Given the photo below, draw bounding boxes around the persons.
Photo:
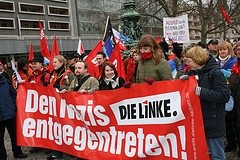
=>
[180,46,230,160]
[123,35,173,88]
[0,35,240,160]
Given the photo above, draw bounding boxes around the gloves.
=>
[123,81,132,88]
[142,78,153,85]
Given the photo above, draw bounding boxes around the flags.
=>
[27,43,35,61]
[49,34,61,74]
[112,28,127,46]
[77,38,85,56]
[221,7,232,25]
[39,20,51,60]
[85,42,103,78]
[102,15,114,42]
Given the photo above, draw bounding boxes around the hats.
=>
[29,57,43,63]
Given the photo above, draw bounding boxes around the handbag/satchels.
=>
[225,95,234,111]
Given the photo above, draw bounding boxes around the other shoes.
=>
[47,154,62,160]
[15,153,27,158]
